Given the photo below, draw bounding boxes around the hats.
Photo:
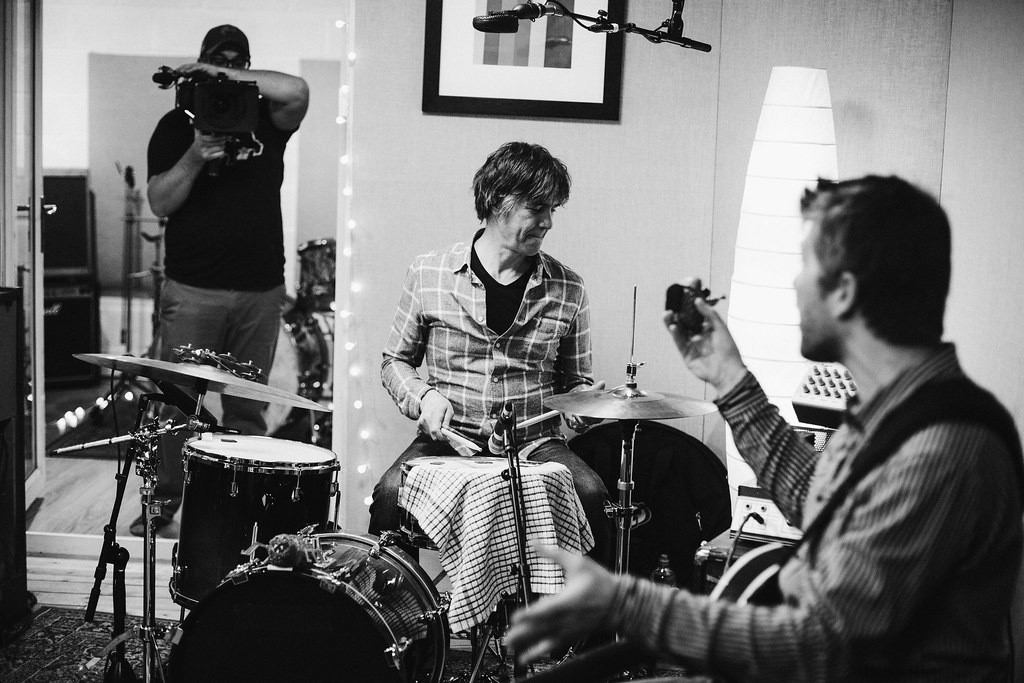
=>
[201,24,249,53]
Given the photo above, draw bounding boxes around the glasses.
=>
[208,56,251,70]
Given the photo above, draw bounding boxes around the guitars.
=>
[505,541,799,683]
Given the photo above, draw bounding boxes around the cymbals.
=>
[72,351,335,414]
[540,384,718,421]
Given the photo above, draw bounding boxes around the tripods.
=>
[66,379,211,683]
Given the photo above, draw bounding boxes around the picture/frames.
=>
[421,0,625,121]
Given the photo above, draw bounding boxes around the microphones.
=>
[473,4,556,33]
[489,400,514,454]
[151,72,173,84]
[152,378,218,425]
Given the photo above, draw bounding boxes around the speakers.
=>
[40,167,102,385]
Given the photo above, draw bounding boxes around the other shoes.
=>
[129,507,174,537]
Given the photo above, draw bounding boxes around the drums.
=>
[295,238,340,308]
[199,290,336,436]
[400,450,567,551]
[163,530,450,683]
[170,431,341,613]
[563,417,731,589]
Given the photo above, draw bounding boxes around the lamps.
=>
[726,66,838,518]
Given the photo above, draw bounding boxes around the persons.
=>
[369,142,618,573]
[500,173,1024,683]
[125,27,312,533]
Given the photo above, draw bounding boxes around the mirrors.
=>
[6,0,356,559]
